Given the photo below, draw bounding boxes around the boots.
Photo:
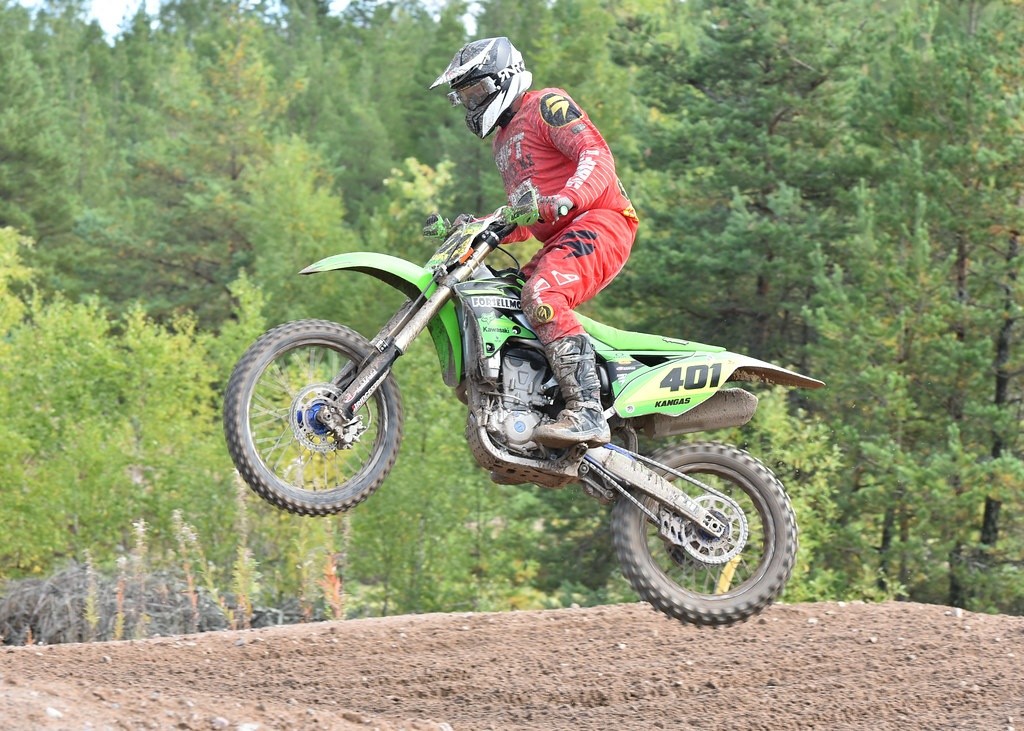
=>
[489,470,529,485]
[534,333,611,450]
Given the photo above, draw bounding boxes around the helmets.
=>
[429,36,532,138]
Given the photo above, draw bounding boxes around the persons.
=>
[430,37,640,449]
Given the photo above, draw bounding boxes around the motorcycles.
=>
[223,204,826,630]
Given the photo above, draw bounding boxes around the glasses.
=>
[447,76,499,108]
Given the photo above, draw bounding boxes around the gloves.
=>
[536,195,574,224]
[452,213,475,226]
